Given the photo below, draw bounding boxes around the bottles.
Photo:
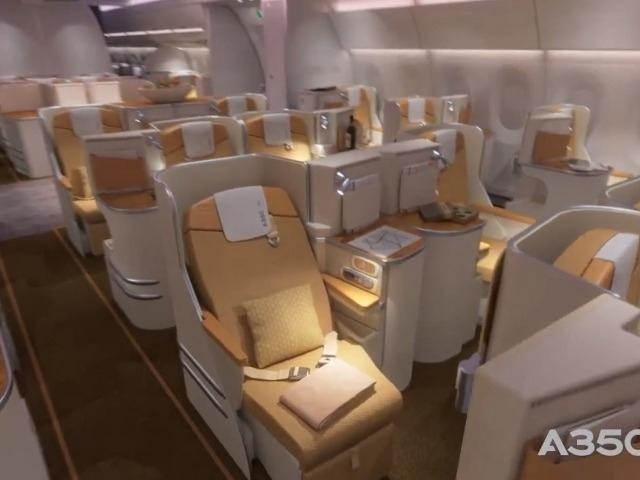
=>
[344,115,356,150]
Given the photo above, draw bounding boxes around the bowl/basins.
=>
[138,85,193,104]
